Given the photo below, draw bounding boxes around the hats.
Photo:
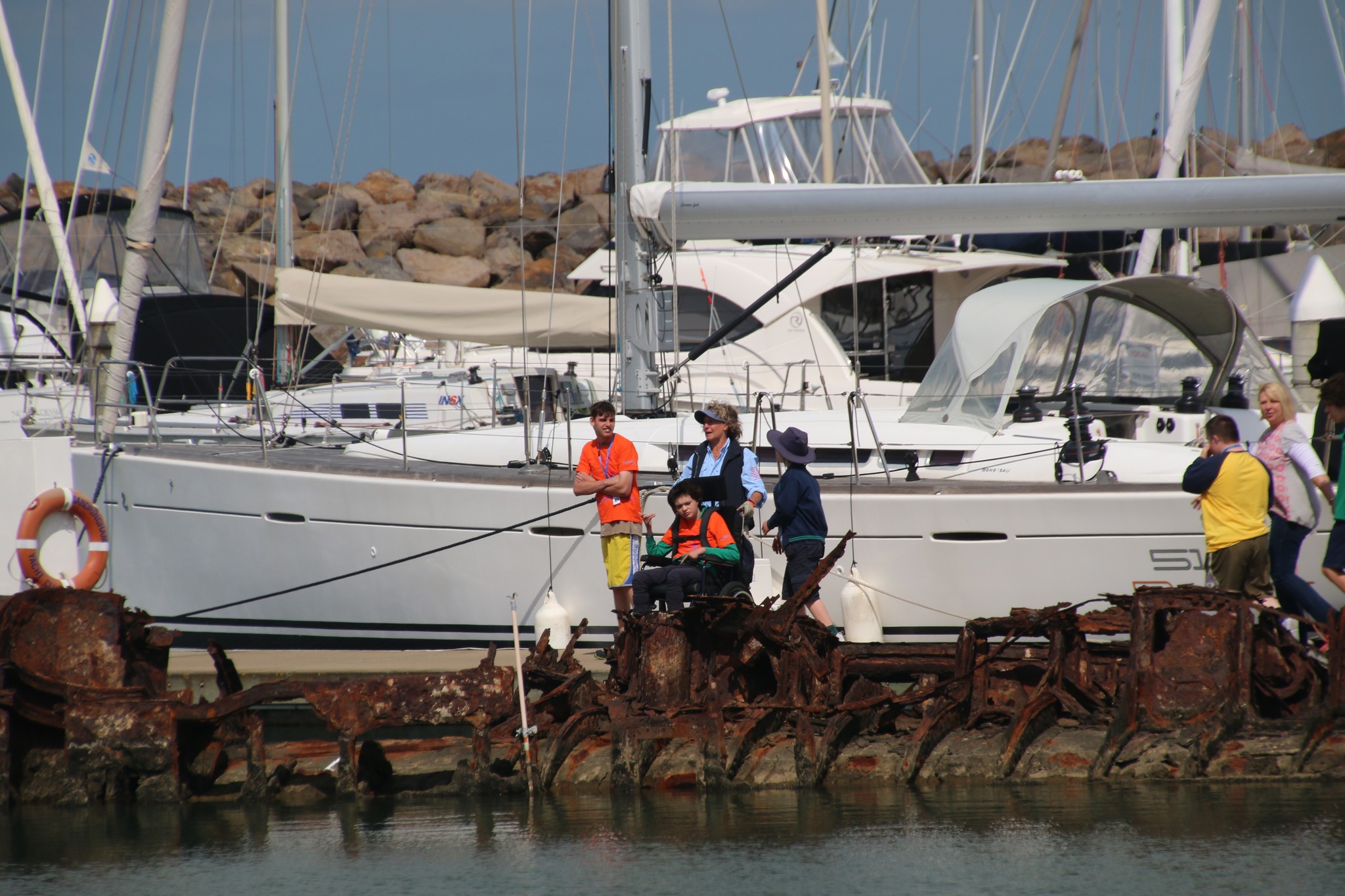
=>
[694,409,726,425]
[766,427,816,464]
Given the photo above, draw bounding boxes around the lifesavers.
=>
[15,487,109,591]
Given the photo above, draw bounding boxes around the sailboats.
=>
[0,1,1345,679]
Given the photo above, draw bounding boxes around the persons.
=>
[1183,415,1289,630]
[1317,372,1345,592]
[573,400,844,639]
[1253,381,1342,653]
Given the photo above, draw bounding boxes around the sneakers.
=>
[594,645,615,659]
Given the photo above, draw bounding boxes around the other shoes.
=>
[1309,626,1330,652]
[836,630,846,642]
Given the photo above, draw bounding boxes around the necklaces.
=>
[711,451,721,457]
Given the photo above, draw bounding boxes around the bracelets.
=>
[1329,495,1336,504]
[747,498,757,507]
